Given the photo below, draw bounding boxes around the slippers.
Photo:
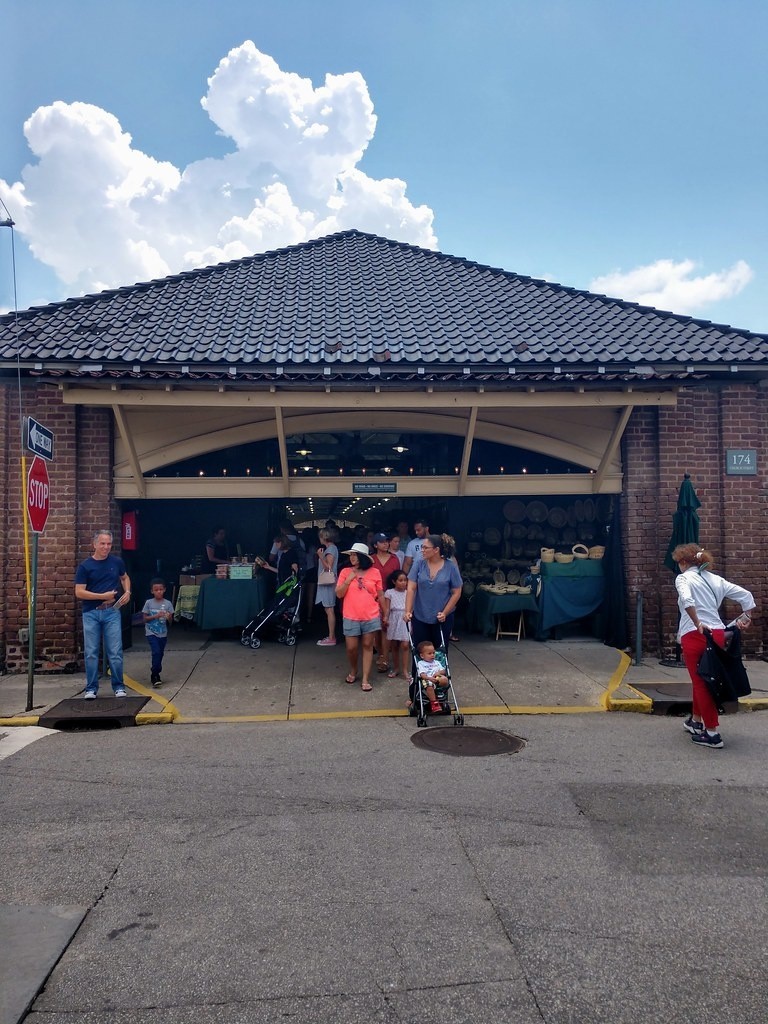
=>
[361,683,372,691]
[346,672,356,683]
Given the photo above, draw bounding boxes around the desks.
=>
[538,560,609,640]
[194,578,272,631]
[476,586,538,642]
[174,586,201,622]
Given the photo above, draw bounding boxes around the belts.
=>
[95,603,113,610]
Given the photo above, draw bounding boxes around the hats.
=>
[341,543,375,564]
[371,533,388,546]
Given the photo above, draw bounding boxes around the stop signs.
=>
[27,455,52,533]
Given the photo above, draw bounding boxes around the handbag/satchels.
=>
[318,566,336,586]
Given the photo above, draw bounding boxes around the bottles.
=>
[230,557,238,565]
[238,553,249,564]
[159,604,166,623]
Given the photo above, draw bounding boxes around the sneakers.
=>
[684,716,703,735]
[115,689,126,697]
[691,728,724,748]
[84,691,97,699]
[316,637,336,646]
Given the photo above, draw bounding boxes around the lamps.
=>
[392,434,410,452]
[295,433,312,455]
[299,454,313,471]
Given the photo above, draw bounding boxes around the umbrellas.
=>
[663,479,701,572]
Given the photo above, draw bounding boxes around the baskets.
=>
[541,547,555,562]
[555,552,574,564]
[572,543,589,558]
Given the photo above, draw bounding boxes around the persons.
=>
[201,525,229,574]
[334,543,387,691]
[141,578,174,686]
[338,520,459,672]
[262,518,338,646]
[417,641,448,712]
[672,543,756,749]
[75,530,130,698]
[385,569,411,682]
[403,533,463,707]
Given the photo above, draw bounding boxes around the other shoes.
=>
[404,675,411,681]
[152,675,162,686]
[449,636,460,642]
[377,656,391,672]
[431,700,442,712]
[388,671,400,677]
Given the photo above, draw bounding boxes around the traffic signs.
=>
[28,416,54,461]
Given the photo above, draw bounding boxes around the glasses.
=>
[421,546,437,550]
[358,578,362,588]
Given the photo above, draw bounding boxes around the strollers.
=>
[408,620,465,728]
[240,568,307,649]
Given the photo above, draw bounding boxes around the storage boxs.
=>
[179,574,211,585]
[216,562,259,579]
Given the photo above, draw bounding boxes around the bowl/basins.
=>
[482,584,519,595]
[255,555,265,566]
[517,587,531,595]
[215,564,228,579]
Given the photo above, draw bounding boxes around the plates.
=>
[520,573,530,587]
[493,571,505,583]
[507,569,521,584]
[484,499,596,545]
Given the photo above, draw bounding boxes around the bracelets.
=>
[698,623,701,628]
[126,591,131,594]
